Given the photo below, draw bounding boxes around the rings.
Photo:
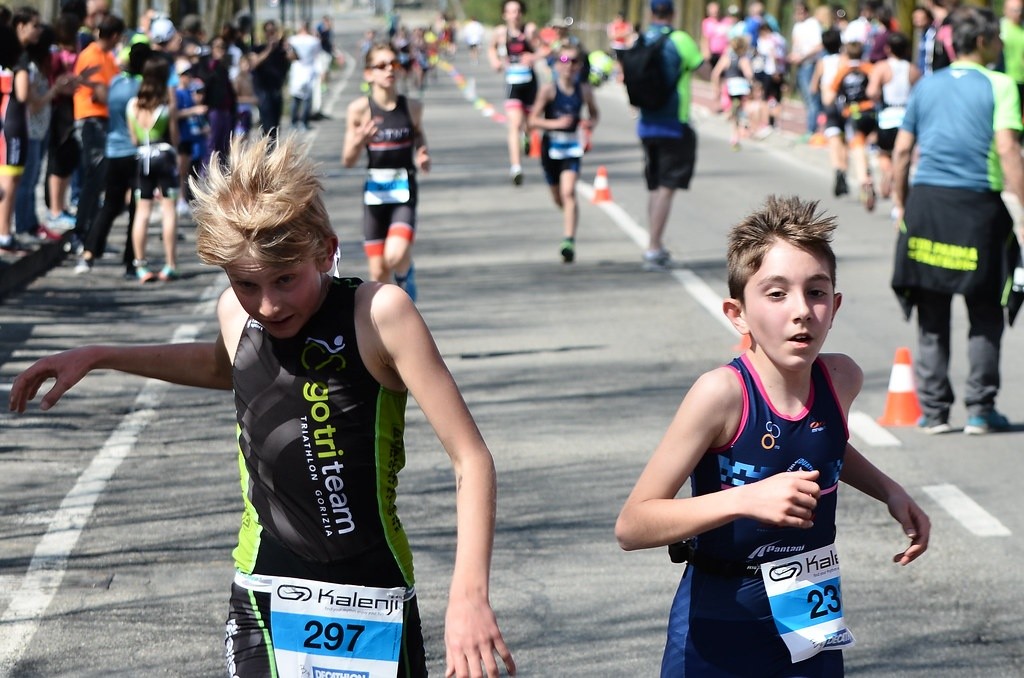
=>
[61,79,68,84]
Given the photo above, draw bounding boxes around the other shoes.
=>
[0,204,78,257]
[917,414,951,435]
[862,180,874,211]
[394,259,417,302]
[835,169,848,197]
[964,409,1011,435]
[559,240,576,262]
[640,249,678,272]
[74,260,95,276]
[135,266,178,283]
[511,166,523,185]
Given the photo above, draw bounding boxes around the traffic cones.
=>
[527,131,542,159]
[875,347,924,424]
[589,165,615,204]
[729,332,752,353]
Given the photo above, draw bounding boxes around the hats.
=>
[652,0,674,13]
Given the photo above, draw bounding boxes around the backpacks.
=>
[616,29,683,107]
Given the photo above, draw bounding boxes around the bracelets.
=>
[0,0,337,284]
[49,86,59,99]
[284,47,294,54]
[76,75,84,82]
[133,258,148,267]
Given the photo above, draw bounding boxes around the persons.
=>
[700,0,1024,211]
[340,41,431,302]
[7,127,517,678]
[637,0,711,271]
[357,0,484,94]
[614,195,931,678]
[889,7,1024,435]
[488,0,600,263]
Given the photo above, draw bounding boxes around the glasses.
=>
[370,58,401,70]
[559,55,579,64]
